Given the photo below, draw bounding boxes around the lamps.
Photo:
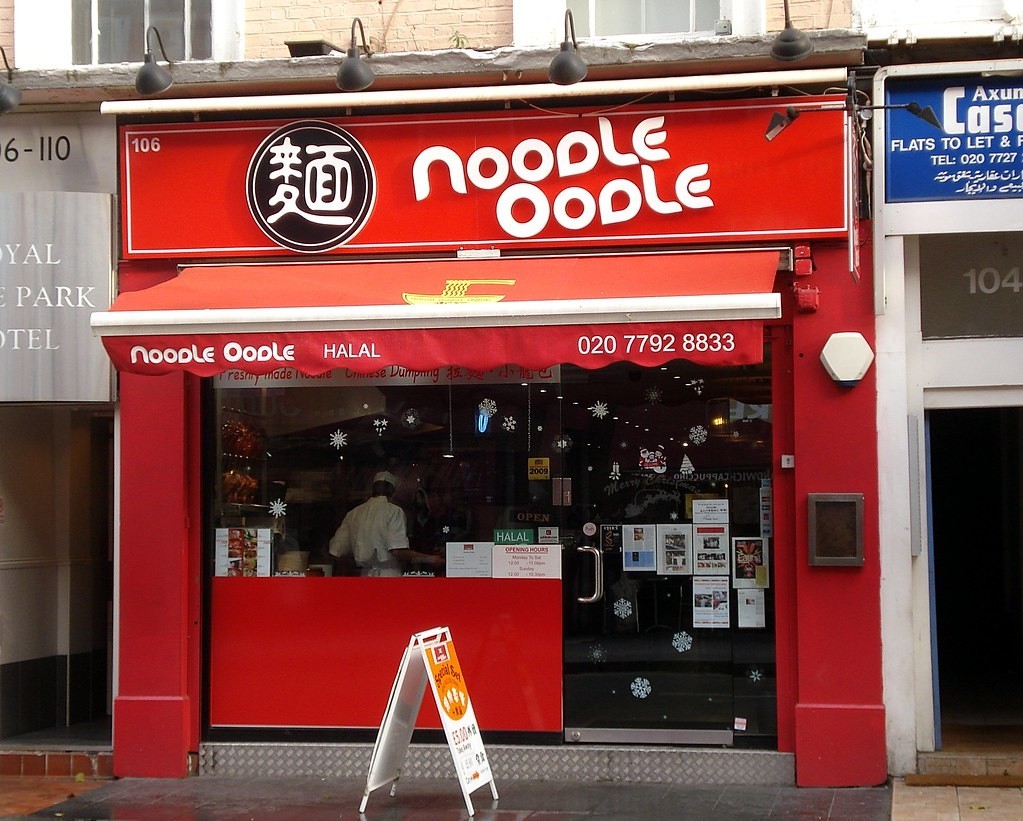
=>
[134,24,175,96]
[335,17,376,91]
[770,0,814,61]
[547,7,588,85]
[0,45,23,116]
[786,102,950,134]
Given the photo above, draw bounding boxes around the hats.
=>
[374,471,397,488]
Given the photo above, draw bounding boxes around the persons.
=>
[328,471,445,576]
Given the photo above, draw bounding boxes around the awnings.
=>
[96,253,787,375]
